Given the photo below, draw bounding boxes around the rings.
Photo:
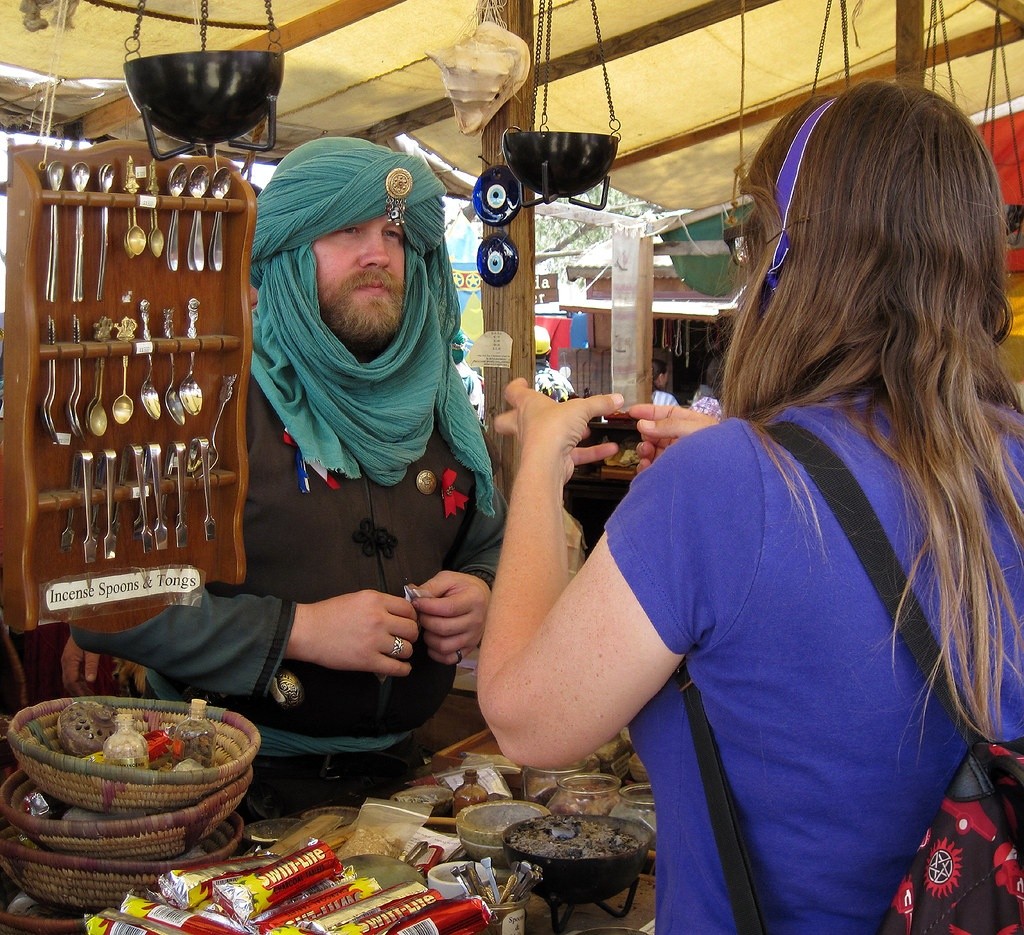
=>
[456,650,463,663]
[387,636,404,658]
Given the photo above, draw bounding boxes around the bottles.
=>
[545,773,622,815]
[452,769,487,819]
[607,782,657,851]
[103,712,149,770]
[585,724,650,782]
[522,760,590,807]
[172,698,218,769]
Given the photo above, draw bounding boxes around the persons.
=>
[478,77,1024,935]
[58,138,510,856]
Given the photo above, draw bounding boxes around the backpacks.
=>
[677,415,1024,935]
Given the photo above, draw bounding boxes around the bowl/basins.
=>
[391,785,452,816]
[456,801,551,867]
[243,818,299,846]
[301,807,359,824]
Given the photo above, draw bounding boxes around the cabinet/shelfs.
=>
[0,0,257,630]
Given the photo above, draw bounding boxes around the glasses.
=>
[724,224,750,268]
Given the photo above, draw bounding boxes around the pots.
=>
[501,814,654,933]
[502,130,618,210]
[124,49,284,161]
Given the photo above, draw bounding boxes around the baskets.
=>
[0,700,261,906]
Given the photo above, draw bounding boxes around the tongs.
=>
[61,435,218,564]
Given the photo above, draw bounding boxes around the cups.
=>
[426,861,496,900]
[476,890,530,935]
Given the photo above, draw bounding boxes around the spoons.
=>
[190,374,241,481]
[42,296,204,446]
[40,161,233,302]
